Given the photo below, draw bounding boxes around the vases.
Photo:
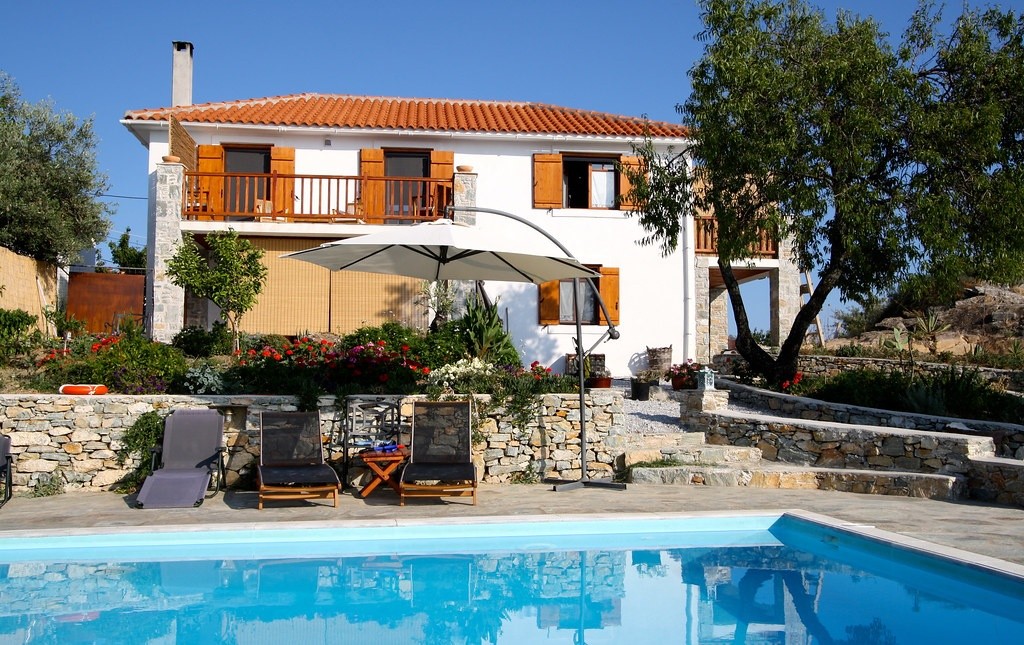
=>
[671,376,698,389]
[630,378,650,401]
[588,377,614,387]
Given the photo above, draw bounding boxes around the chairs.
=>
[255,408,342,510]
[252,198,293,221]
[334,202,364,225]
[133,408,227,510]
[399,399,478,506]
[0,433,14,509]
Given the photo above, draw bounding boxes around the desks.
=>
[187,189,223,221]
[410,195,437,225]
[356,444,412,500]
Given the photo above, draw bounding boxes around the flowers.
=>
[665,358,705,381]
